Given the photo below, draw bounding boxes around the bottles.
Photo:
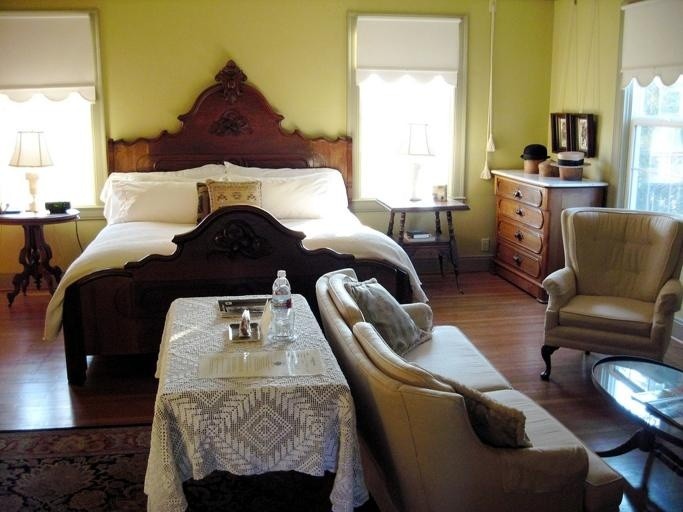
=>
[272,270,291,292]
[273,278,292,310]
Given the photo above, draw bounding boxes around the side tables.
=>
[376,198,469,293]
[1,209,81,306]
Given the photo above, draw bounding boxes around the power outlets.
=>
[481,239,490,250]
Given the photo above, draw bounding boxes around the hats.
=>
[521,144,550,160]
[549,152,591,168]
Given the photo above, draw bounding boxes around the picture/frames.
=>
[572,114,595,158]
[550,113,570,153]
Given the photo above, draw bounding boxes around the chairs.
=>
[541,207,682,380]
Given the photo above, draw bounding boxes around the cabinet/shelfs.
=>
[491,169,609,303]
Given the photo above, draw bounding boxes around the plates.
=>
[228,322,261,343]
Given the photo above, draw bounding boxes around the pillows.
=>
[354,320,454,392]
[196,183,210,223]
[228,176,321,219]
[224,161,348,210]
[437,374,531,446]
[207,180,261,216]
[111,179,206,224]
[346,276,431,355]
[100,164,223,222]
[331,273,364,327]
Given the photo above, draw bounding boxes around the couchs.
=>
[314,267,624,512]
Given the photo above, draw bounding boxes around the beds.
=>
[42,59,433,383]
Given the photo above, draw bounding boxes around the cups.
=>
[271,308,296,342]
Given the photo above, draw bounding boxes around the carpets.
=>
[0,422,391,511]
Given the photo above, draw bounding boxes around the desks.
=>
[142,294,368,502]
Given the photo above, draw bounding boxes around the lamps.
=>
[8,131,53,213]
